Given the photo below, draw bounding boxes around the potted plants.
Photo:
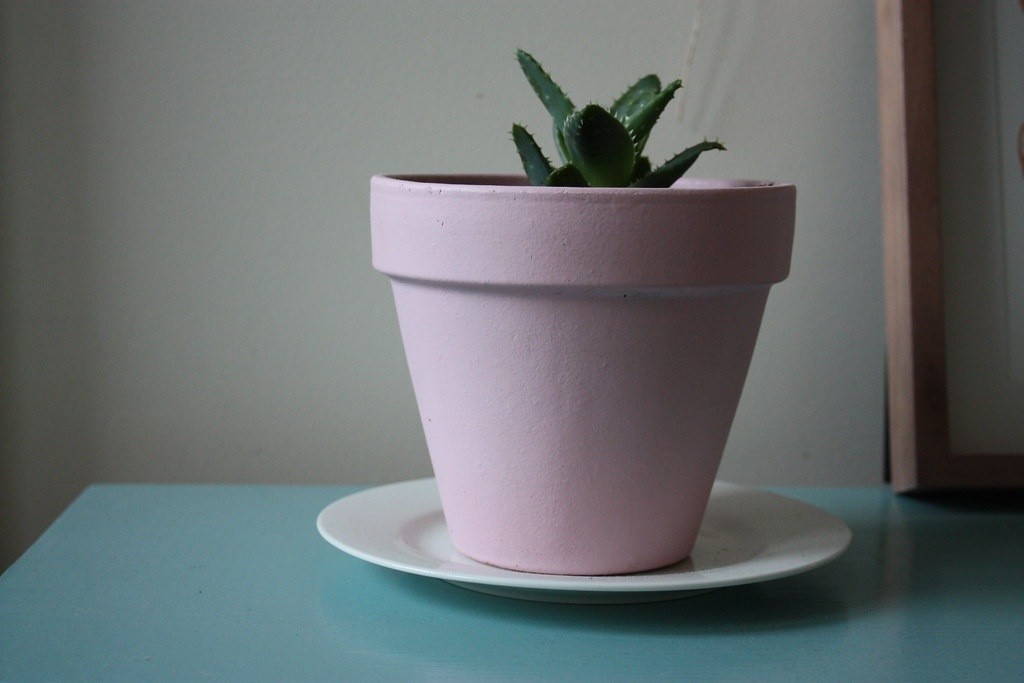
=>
[367,48,798,577]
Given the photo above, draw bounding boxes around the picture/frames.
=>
[880,0,1024,494]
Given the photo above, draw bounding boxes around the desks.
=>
[0,476,1024,683]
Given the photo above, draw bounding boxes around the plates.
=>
[317,476,855,606]
